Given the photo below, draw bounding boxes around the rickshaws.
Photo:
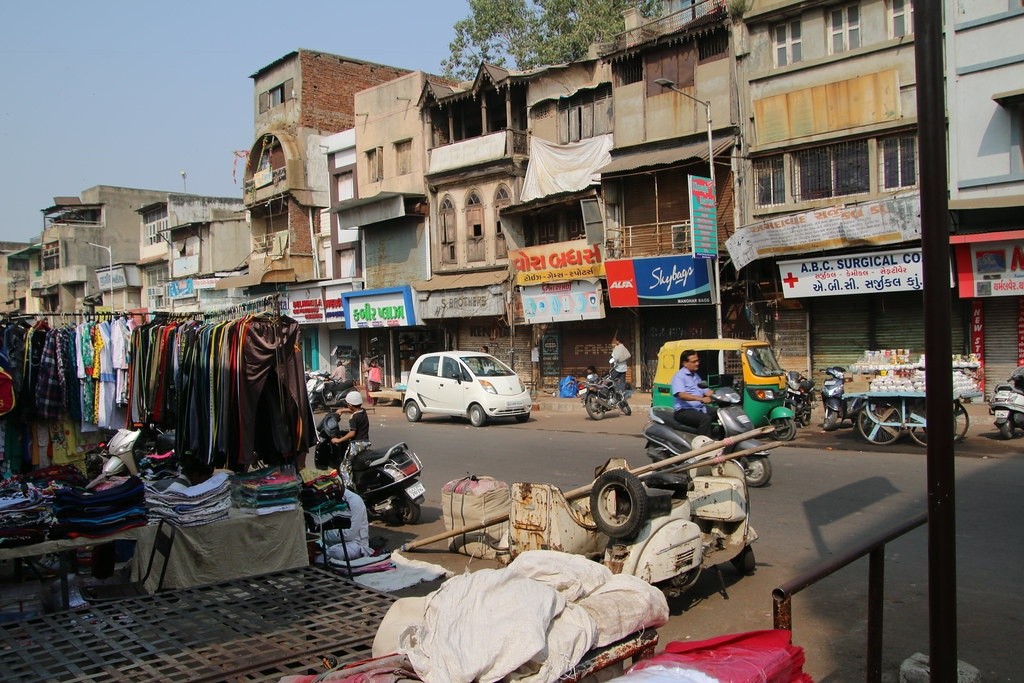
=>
[841,390,983,448]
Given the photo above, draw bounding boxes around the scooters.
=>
[603,433,761,605]
[641,383,772,488]
[987,365,1024,439]
[314,412,425,524]
[819,366,855,430]
[305,371,356,411]
[102,427,176,480]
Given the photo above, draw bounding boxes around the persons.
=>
[362,359,382,406]
[609,335,627,384]
[480,346,489,352]
[670,350,715,437]
[331,391,371,491]
[331,361,346,380]
[582,366,596,378]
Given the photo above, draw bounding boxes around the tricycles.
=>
[649,337,797,443]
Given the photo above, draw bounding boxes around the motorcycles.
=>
[779,368,815,425]
[575,357,631,420]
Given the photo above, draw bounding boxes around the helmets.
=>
[337,362,342,364]
[345,390,363,406]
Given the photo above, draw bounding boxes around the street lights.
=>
[654,79,725,378]
[80,240,114,313]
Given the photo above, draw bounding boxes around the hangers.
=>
[0,312,135,330]
[151,294,282,326]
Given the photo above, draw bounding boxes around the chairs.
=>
[79,519,176,603]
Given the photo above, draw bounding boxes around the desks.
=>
[0,504,353,610]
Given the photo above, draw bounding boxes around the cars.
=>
[402,350,533,426]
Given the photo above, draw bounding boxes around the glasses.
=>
[690,358,699,363]
[481,348,482,350]
